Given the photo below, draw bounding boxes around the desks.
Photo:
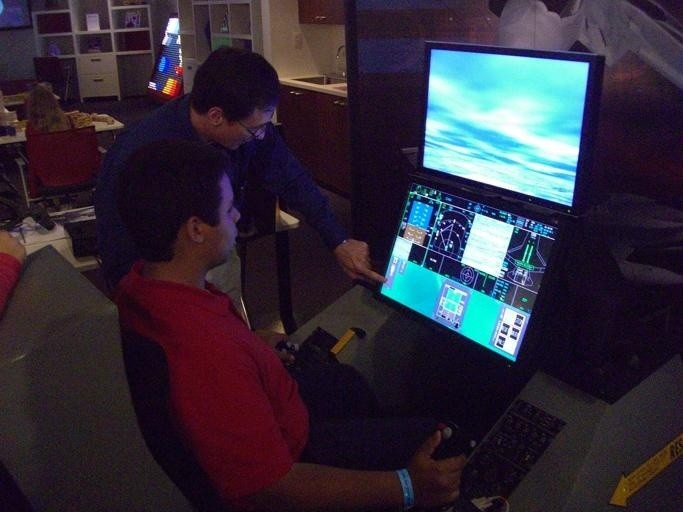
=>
[0,112,124,149]
[1,90,62,108]
[291,280,681,510]
[12,178,296,334]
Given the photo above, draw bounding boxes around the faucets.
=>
[335,45,347,78]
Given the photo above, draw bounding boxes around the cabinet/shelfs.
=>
[314,91,348,197]
[274,84,315,183]
[297,0,346,24]
[30,0,157,103]
[177,0,269,94]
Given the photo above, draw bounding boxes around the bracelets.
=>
[392,465,418,510]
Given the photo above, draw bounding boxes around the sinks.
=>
[292,76,348,85]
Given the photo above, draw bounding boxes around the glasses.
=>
[236,119,268,139]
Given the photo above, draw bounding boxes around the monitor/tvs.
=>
[373,171,564,368]
[417,40,606,214]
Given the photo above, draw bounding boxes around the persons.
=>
[0,229,25,321]
[93,44,385,330]
[103,138,467,511]
[25,82,74,206]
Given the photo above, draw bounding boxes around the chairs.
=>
[0,251,211,512]
[33,55,75,102]
[8,112,108,214]
